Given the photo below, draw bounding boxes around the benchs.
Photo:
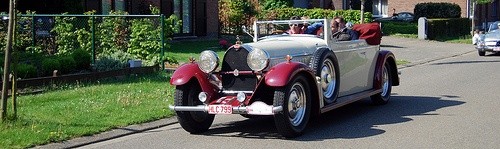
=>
[312,24,382,44]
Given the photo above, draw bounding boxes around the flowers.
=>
[220,40,233,52]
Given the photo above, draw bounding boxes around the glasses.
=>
[289,23,298,27]
[303,26,308,28]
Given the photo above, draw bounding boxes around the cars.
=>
[168,17,400,139]
[373,12,417,24]
[475,21,500,56]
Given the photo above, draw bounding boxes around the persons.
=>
[282,15,359,41]
[472,29,485,45]
[392,9,398,20]
